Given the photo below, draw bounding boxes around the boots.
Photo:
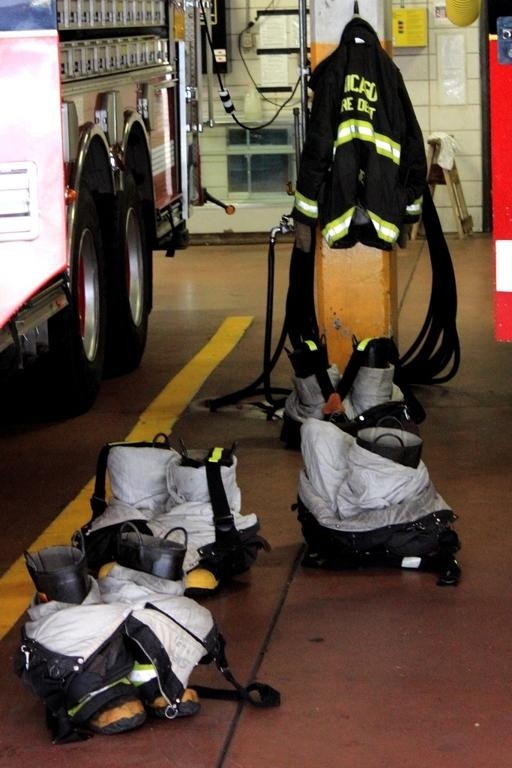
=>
[113,522,202,717]
[95,433,178,581]
[174,437,239,597]
[278,333,460,571]
[22,531,147,734]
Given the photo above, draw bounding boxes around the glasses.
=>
[435,528,460,588]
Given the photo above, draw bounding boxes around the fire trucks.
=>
[0,1,234,425]
